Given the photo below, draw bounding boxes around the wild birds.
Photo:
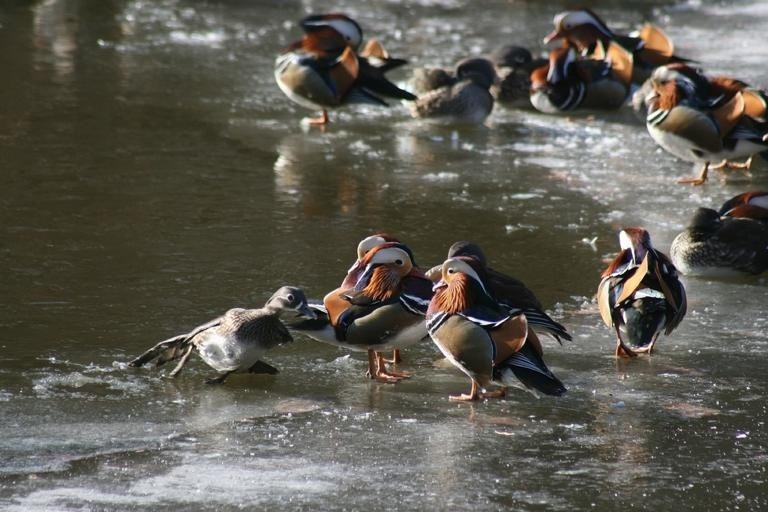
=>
[285,235,434,405]
[127,284,321,386]
[425,241,574,406]
[271,8,768,185]
[597,227,688,359]
[670,191,767,280]
